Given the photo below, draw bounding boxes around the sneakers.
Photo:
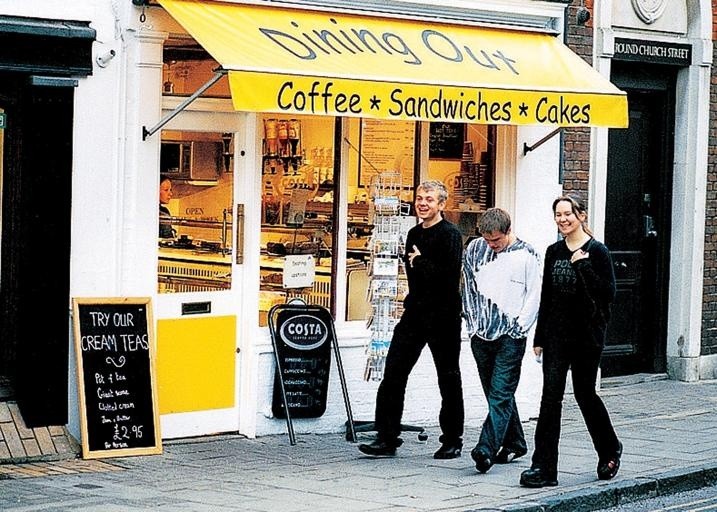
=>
[520,464,558,486]
[597,441,622,479]
[358,442,396,458]
[493,446,526,464]
[471,448,494,473]
[434,444,462,459]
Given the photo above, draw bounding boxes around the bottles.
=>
[261,118,301,175]
[312,145,334,190]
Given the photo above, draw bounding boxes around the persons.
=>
[460,207,543,475]
[519,191,623,489]
[357,180,464,460]
[159,174,174,238]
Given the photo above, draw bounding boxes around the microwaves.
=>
[161,138,224,182]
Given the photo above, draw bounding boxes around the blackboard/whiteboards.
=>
[72,298,164,460]
[429,122,468,161]
[272,309,332,419]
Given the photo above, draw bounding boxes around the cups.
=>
[453,141,487,212]
